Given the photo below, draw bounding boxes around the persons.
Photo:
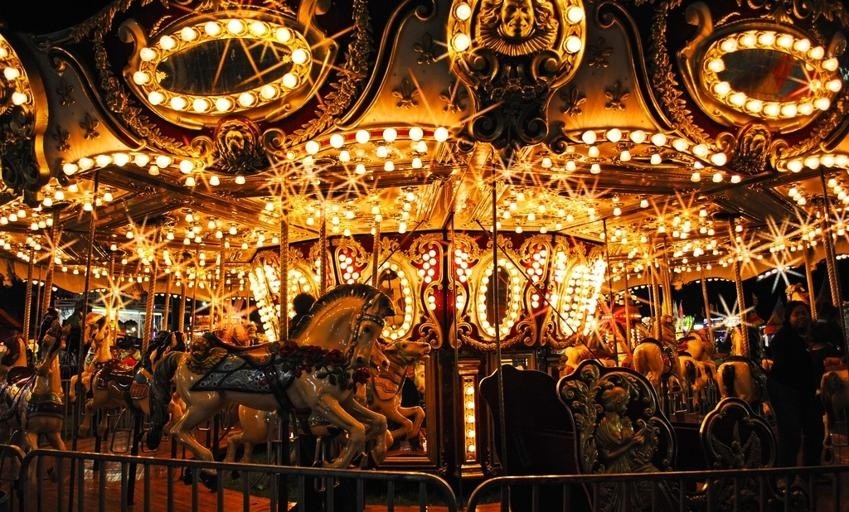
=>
[289,291,316,334]
[661,314,680,352]
[718,309,761,364]
[62,296,93,361]
[763,301,849,490]
[480,0,554,42]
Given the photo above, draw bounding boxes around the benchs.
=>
[476,358,774,512]
